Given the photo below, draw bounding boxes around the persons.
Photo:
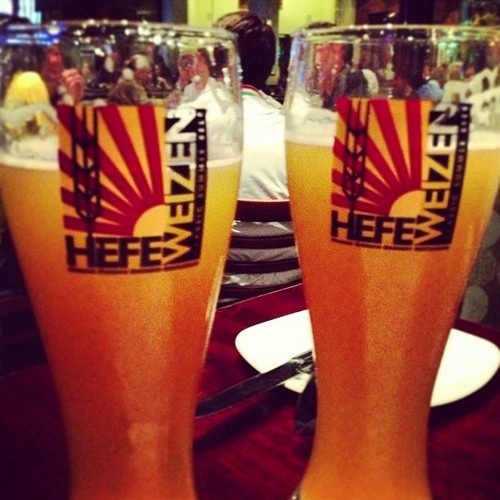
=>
[0,12,500,287]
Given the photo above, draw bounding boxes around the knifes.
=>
[195,352,312,419]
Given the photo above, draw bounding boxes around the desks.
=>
[0,283,500,499]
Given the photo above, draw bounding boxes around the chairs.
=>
[217,200,303,308]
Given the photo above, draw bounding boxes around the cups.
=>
[0,22,245,500]
[287,25,500,499]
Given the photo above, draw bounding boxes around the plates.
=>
[234,306,500,408]
